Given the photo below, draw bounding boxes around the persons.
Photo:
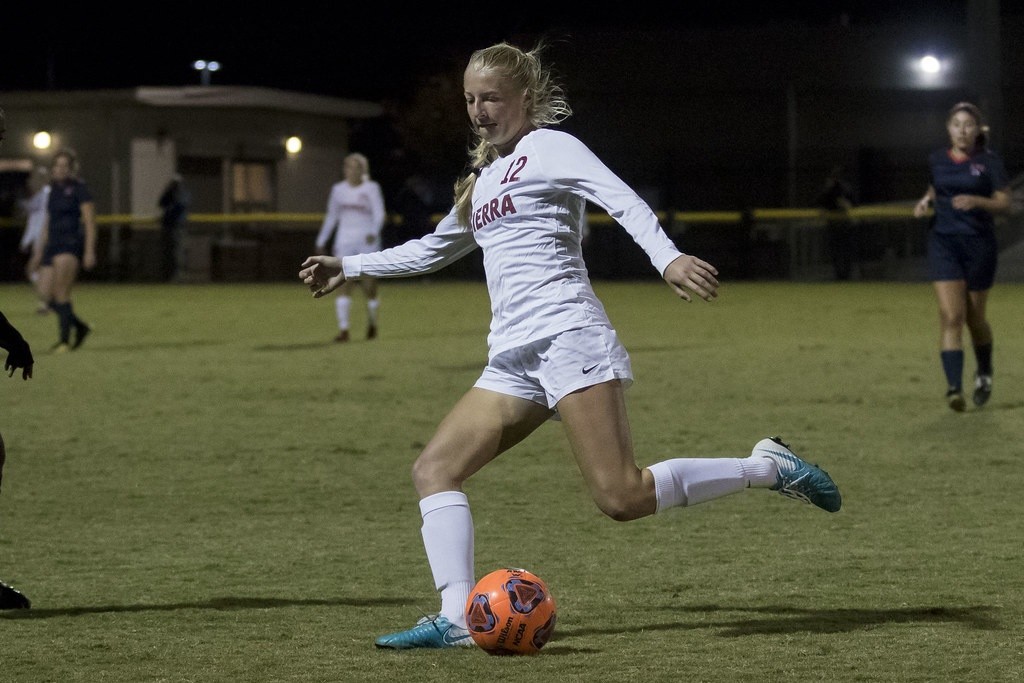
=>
[915,102,1013,413]
[0,311,34,614]
[21,149,98,353]
[316,154,385,343]
[299,47,844,649]
[154,174,191,284]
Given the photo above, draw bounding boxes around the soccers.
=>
[464,568,556,655]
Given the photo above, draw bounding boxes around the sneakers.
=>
[973,375,993,406]
[375,614,478,650]
[751,437,842,513]
[948,394,967,413]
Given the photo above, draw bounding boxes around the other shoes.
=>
[0,582,32,610]
[53,322,91,352]
[367,326,378,340]
[333,330,350,342]
